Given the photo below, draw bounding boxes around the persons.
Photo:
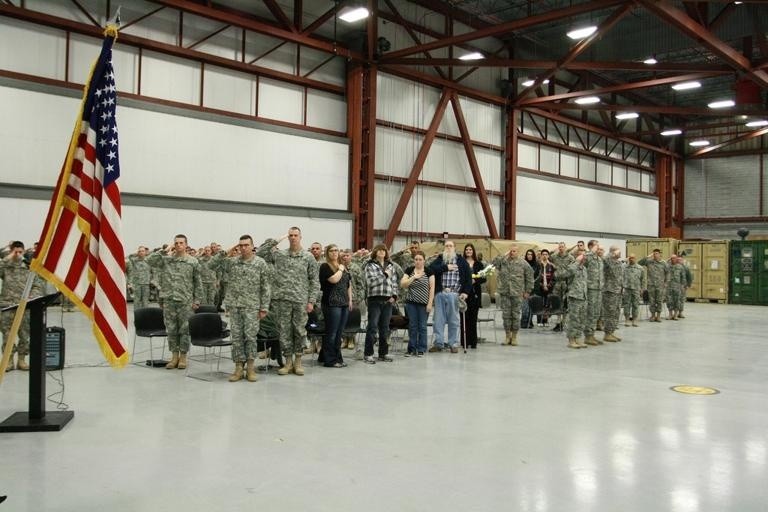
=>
[0,241,82,378]
[429,241,472,353]
[318,244,352,368]
[459,243,487,349]
[207,234,271,380]
[520,240,692,349]
[126,240,429,359]
[256,227,320,375]
[144,234,204,369]
[362,243,399,363]
[400,251,435,357]
[491,244,535,346]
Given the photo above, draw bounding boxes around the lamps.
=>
[185,313,232,382]
[639,289,650,318]
[389,305,407,352]
[343,308,367,358]
[132,308,168,367]
[494,293,503,321]
[477,318,497,342]
[544,295,563,330]
[195,305,230,339]
[527,295,543,328]
[305,304,325,367]
[481,292,502,326]
[259,337,278,374]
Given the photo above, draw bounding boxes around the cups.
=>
[411,247,417,249]
[329,249,337,252]
[578,245,582,246]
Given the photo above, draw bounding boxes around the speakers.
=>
[229,363,244,382]
[246,363,257,382]
[294,353,305,375]
[278,355,293,375]
[166,351,179,369]
[16,354,29,370]
[178,353,187,369]
[341,338,347,348]
[6,356,14,372]
[348,338,355,349]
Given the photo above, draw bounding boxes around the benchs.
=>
[30,26,131,370]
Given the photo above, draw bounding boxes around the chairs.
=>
[418,352,423,357]
[404,353,412,357]
[625,317,631,326]
[651,313,656,322]
[605,332,618,342]
[378,355,392,362]
[568,338,581,348]
[679,311,684,318]
[584,335,598,345]
[592,335,603,344]
[611,333,621,341]
[451,348,458,353]
[502,331,511,345]
[673,310,679,320]
[364,356,376,364]
[511,332,518,346]
[632,317,638,327]
[666,310,673,320]
[429,347,441,352]
[304,342,316,354]
[576,338,587,348]
[656,313,661,322]
[315,340,322,354]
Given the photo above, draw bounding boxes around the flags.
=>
[30,26,131,370]
